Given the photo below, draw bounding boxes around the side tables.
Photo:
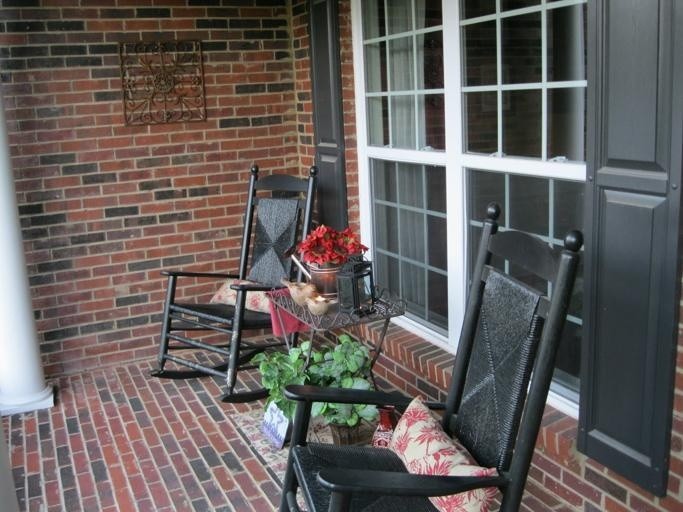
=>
[263,285,405,441]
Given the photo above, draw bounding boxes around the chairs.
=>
[277,201,584,512]
[148,163,318,400]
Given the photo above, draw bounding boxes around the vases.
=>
[308,260,347,299]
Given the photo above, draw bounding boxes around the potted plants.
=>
[249,336,377,445]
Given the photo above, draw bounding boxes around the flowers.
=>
[293,223,369,267]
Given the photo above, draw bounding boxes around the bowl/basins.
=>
[290,284,318,306]
[307,301,330,317]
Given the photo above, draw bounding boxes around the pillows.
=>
[208,278,286,316]
[387,394,506,512]
